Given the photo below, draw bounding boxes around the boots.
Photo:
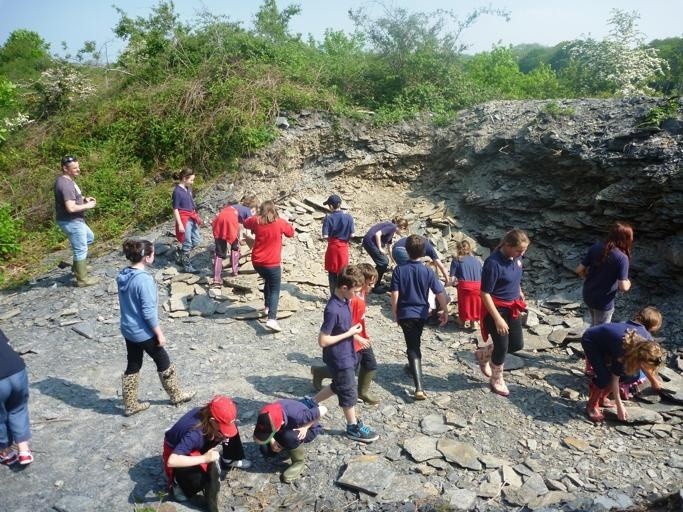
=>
[122,372,150,416]
[157,363,197,405]
[358,367,380,405]
[213,257,223,284]
[585,383,614,422]
[406,357,427,400]
[176,249,200,274]
[231,250,239,275]
[283,442,305,480]
[476,343,510,396]
[311,365,334,391]
[71,259,99,287]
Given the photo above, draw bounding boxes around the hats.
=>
[61,157,73,167]
[322,195,341,206]
[210,396,238,438]
[253,404,283,445]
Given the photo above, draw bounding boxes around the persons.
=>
[171,169,293,331]
[577,221,663,425]
[53,156,101,287]
[116,239,199,416]
[159,398,245,511]
[253,397,325,484]
[0,324,34,468]
[319,196,530,442]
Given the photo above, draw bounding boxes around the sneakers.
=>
[172,479,188,502]
[346,418,380,442]
[18,449,33,465]
[265,310,281,331]
[0,451,17,465]
[221,458,252,468]
